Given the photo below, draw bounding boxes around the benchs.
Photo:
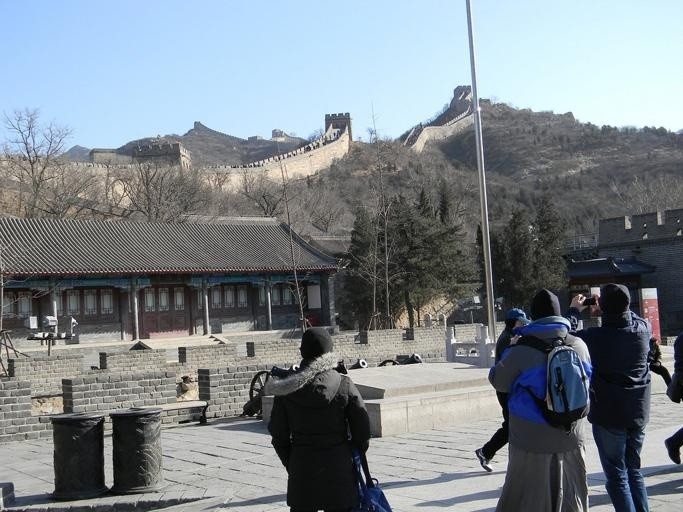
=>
[129,400,210,425]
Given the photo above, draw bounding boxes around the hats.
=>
[299,328,332,357]
[529,288,562,317]
[505,309,529,324]
[601,282,630,311]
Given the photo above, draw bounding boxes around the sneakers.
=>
[475,446,492,473]
[663,438,681,464]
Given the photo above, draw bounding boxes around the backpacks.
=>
[512,337,588,424]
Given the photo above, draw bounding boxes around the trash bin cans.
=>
[109,407,167,494]
[49,412,109,500]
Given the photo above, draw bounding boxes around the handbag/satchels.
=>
[362,484,393,512]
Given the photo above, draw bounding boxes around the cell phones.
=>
[583,298,596,306]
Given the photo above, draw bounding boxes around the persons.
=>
[647,336,671,388]
[487,286,591,512]
[662,330,683,466]
[474,307,530,472]
[267,327,372,511]
[564,281,655,511]
